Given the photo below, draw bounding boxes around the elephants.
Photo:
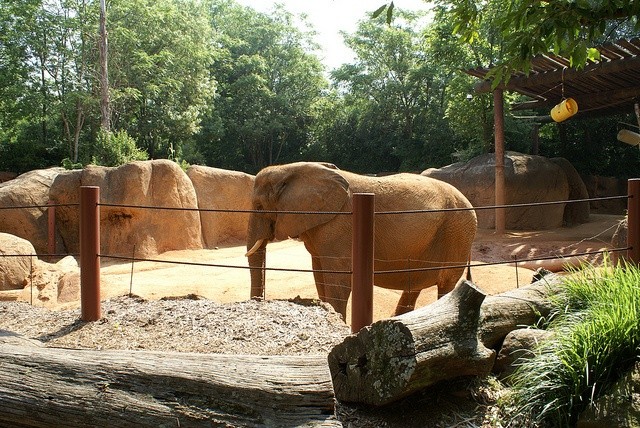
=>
[245,161,477,324]
